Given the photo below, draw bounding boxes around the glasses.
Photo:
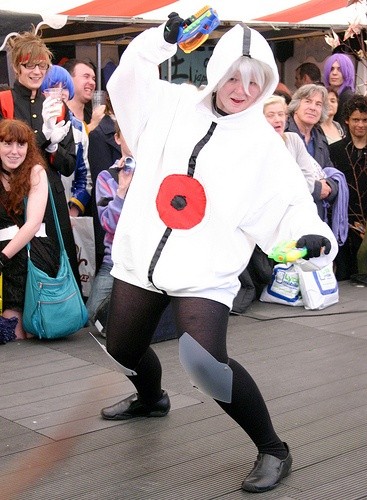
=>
[21,61,50,71]
[265,112,285,118]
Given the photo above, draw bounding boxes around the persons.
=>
[106,12,339,492]
[0,31,367,341]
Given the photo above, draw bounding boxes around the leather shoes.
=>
[241,441,293,494]
[100,389,171,419]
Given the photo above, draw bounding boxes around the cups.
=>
[92,90,105,110]
[43,87,62,106]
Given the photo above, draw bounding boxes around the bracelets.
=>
[68,198,85,215]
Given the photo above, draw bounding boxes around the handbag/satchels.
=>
[22,248,90,339]
[296,259,340,310]
[259,260,304,307]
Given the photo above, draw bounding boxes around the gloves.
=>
[42,97,62,141]
[45,119,72,153]
[296,235,331,260]
[164,12,186,44]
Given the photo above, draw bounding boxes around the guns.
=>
[268,238,325,264]
[166,4,221,55]
